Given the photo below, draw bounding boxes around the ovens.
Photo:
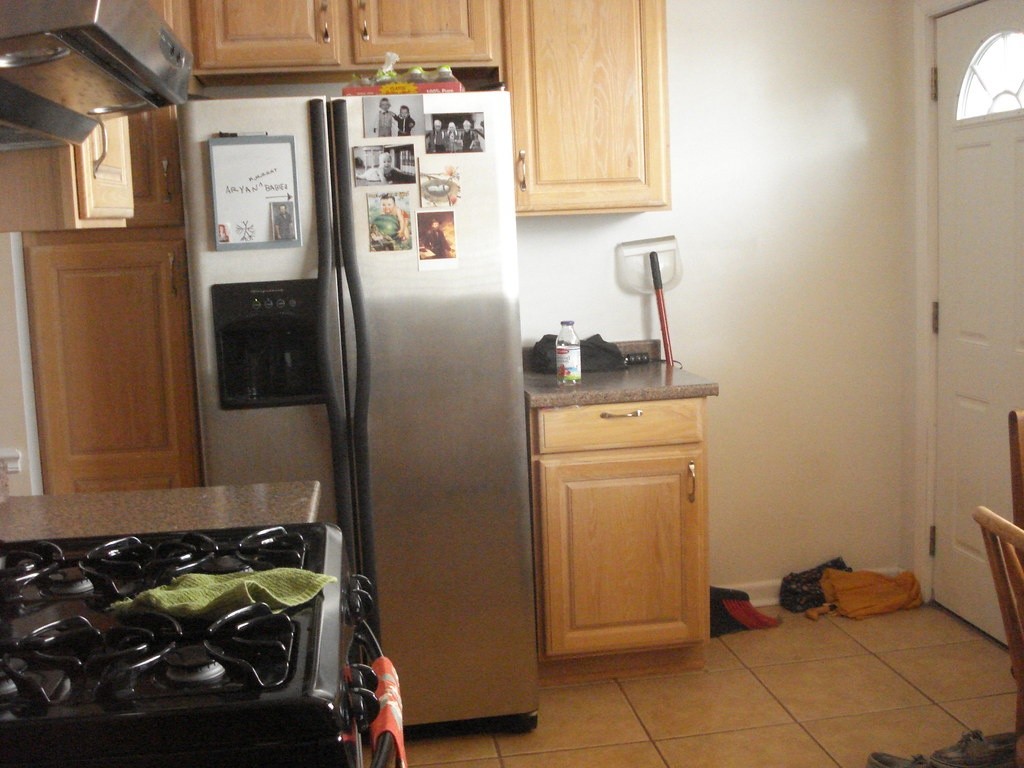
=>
[0,526,410,768]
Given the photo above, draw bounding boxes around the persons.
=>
[425,120,484,151]
[357,152,401,184]
[274,205,294,239]
[425,220,451,257]
[219,225,228,242]
[374,98,415,137]
[379,195,410,242]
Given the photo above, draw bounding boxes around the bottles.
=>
[350,64,456,86]
[555,321,582,391]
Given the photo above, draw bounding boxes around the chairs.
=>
[972,505,1024,768]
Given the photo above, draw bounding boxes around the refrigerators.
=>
[177,81,539,737]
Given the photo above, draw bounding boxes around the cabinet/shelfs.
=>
[128,0,183,229]
[502,0,671,215]
[191,1,494,70]
[28,239,200,496]
[536,398,704,658]
[1,115,134,232]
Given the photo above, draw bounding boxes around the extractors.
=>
[0,0,196,151]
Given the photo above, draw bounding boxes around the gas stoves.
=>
[0,523,330,713]
